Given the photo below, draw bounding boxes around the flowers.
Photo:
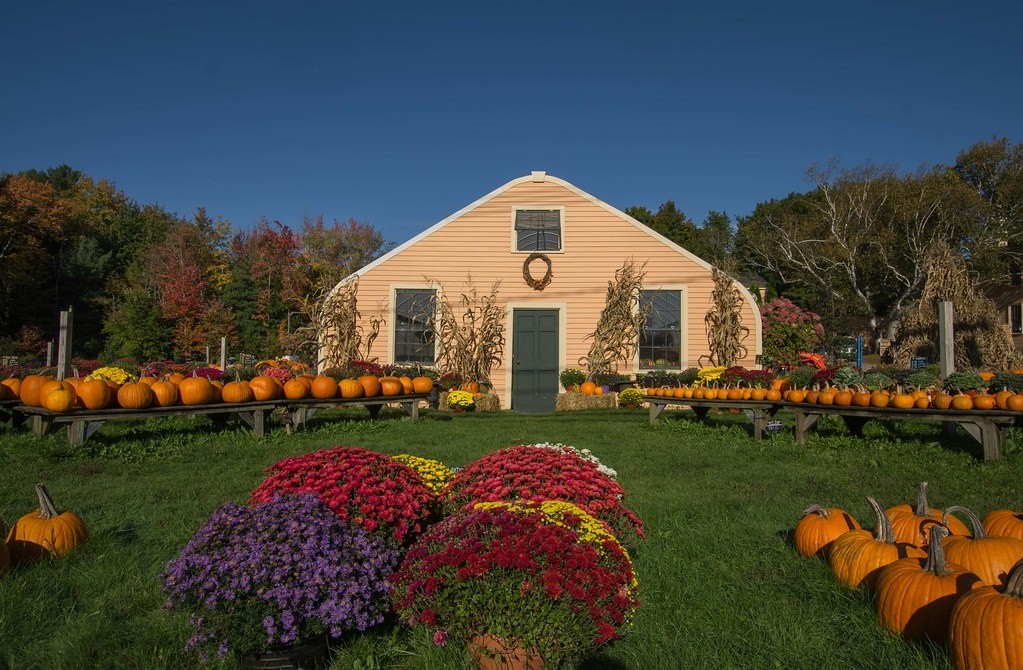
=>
[618,387,646,408]
[446,390,475,410]
[155,442,642,670]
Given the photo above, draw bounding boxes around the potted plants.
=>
[559,368,586,392]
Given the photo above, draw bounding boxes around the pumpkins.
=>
[462,379,478,393]
[0,363,433,412]
[572,381,603,395]
[0,483,88,576]
[647,379,788,400]
[783,382,1023,411]
[795,481,1023,670]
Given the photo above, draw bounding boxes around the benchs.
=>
[0,390,432,449]
[642,395,1023,465]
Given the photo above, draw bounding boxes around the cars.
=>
[838,336,856,360]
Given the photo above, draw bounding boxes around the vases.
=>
[452,407,464,413]
[626,403,637,408]
[232,630,325,670]
[466,634,563,670]
